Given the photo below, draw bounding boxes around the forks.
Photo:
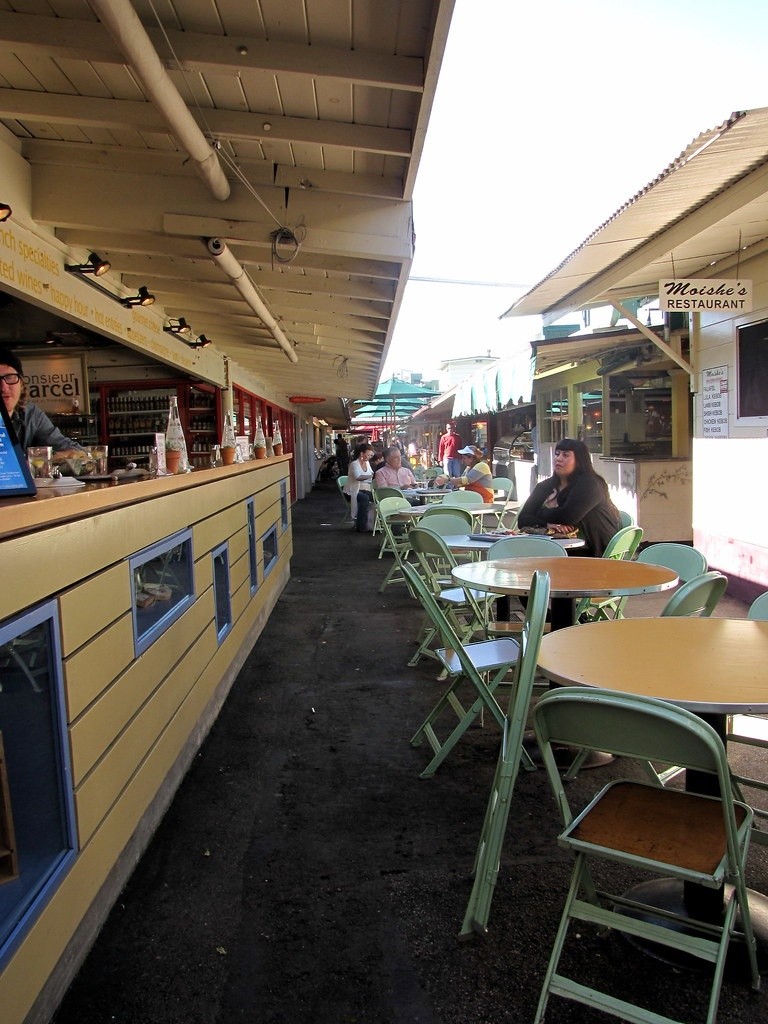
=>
[52,468,60,479]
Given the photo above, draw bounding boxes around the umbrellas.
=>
[354,373,442,447]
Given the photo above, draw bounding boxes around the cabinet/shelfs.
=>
[44,378,223,458]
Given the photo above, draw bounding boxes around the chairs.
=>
[337,465,768,1024]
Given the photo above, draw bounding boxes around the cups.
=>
[28,446,53,478]
[87,445,108,476]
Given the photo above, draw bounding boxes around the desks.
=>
[442,533,585,682]
[401,488,453,503]
[450,557,678,770]
[537,615,768,979]
[400,500,520,516]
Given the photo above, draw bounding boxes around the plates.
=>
[77,474,111,480]
[33,475,85,487]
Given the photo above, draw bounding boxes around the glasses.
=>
[0,373,21,385]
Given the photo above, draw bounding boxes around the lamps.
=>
[163,318,191,334]
[0,203,12,222]
[120,286,156,306]
[189,334,211,348]
[65,252,111,277]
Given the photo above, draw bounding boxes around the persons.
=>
[438,424,463,489]
[343,443,420,544]
[334,434,348,476]
[0,348,82,459]
[518,439,622,624]
[435,445,494,503]
[408,439,422,460]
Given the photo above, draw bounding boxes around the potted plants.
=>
[166,441,180,474]
[272,442,282,456]
[220,445,236,465]
[253,441,266,459]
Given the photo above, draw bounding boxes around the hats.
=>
[457,446,474,455]
[0,347,23,376]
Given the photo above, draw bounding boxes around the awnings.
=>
[451,324,669,419]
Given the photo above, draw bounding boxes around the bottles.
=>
[165,396,189,474]
[252,416,266,449]
[107,395,217,456]
[221,411,238,464]
[271,420,283,456]
